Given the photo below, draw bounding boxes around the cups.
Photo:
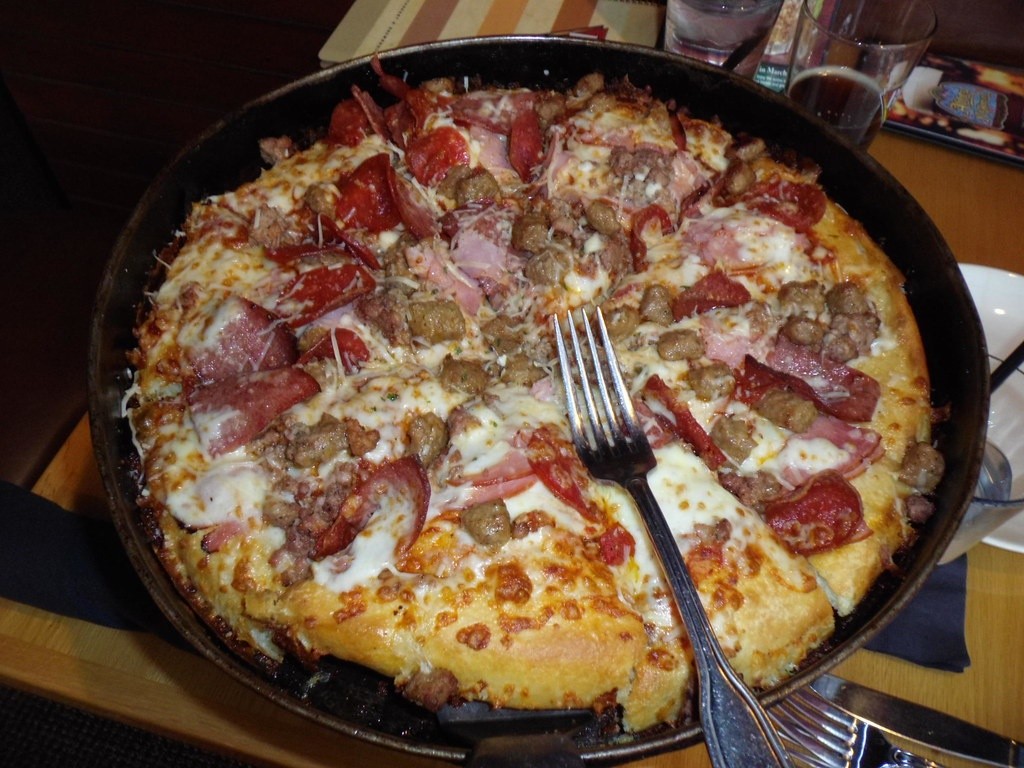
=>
[937,353,1024,566]
[663,0,786,80]
[784,0,939,154]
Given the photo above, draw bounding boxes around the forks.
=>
[767,688,947,768]
[553,305,797,768]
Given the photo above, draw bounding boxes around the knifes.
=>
[807,671,1024,768]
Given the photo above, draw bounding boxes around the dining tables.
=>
[0,133,1024,768]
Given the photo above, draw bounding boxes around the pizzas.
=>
[129,48,945,735]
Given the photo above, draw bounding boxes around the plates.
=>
[85,33,992,768]
[958,262,1024,555]
